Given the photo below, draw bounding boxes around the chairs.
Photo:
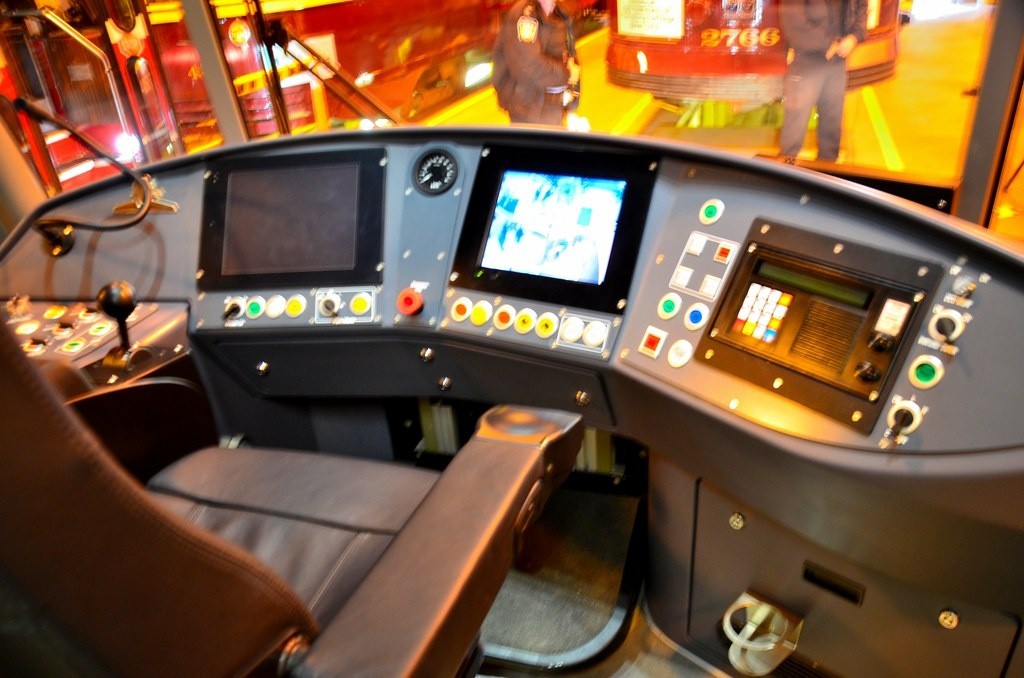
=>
[0,309,584,678]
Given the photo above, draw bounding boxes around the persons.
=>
[779,0,868,162]
[494,0,580,126]
